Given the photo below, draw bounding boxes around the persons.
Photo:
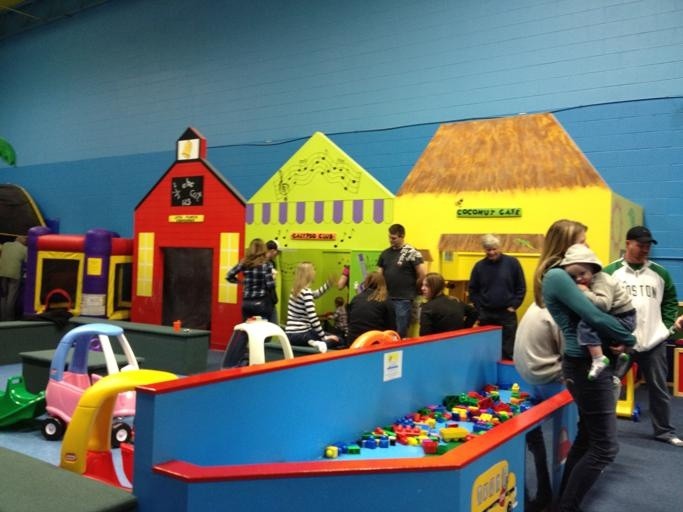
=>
[337,264,350,304]
[530,216,636,512]
[596,227,683,450]
[261,238,279,326]
[283,260,344,345]
[418,272,478,336]
[513,297,573,385]
[340,270,396,349]
[375,223,425,339]
[322,296,348,337]
[226,237,278,321]
[468,232,526,361]
[560,244,636,382]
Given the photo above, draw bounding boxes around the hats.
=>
[627,226,657,245]
[560,244,601,275]
